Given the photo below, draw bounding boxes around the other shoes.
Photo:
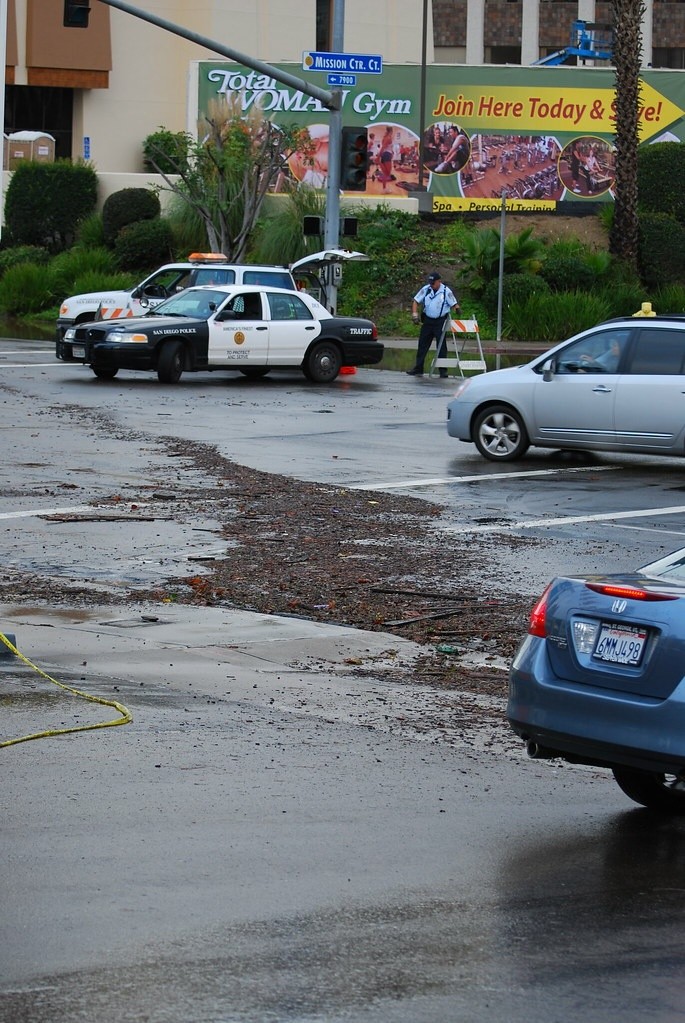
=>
[406,367,424,376]
[439,370,448,378]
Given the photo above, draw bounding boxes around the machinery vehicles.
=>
[530,19,612,67]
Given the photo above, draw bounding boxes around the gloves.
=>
[456,309,461,315]
[412,313,420,325]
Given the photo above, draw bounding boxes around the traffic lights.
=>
[62,0,91,29]
[341,126,370,191]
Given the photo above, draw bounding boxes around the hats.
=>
[428,273,441,282]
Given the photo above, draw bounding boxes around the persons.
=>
[571,139,586,193]
[429,127,445,160]
[584,150,602,194]
[376,144,380,172]
[406,273,462,378]
[400,142,420,179]
[367,133,375,178]
[435,126,470,173]
[374,126,394,193]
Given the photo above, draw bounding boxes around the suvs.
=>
[58,247,373,339]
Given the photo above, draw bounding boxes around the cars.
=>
[503,548,685,812]
[59,282,386,386]
[444,302,685,463]
